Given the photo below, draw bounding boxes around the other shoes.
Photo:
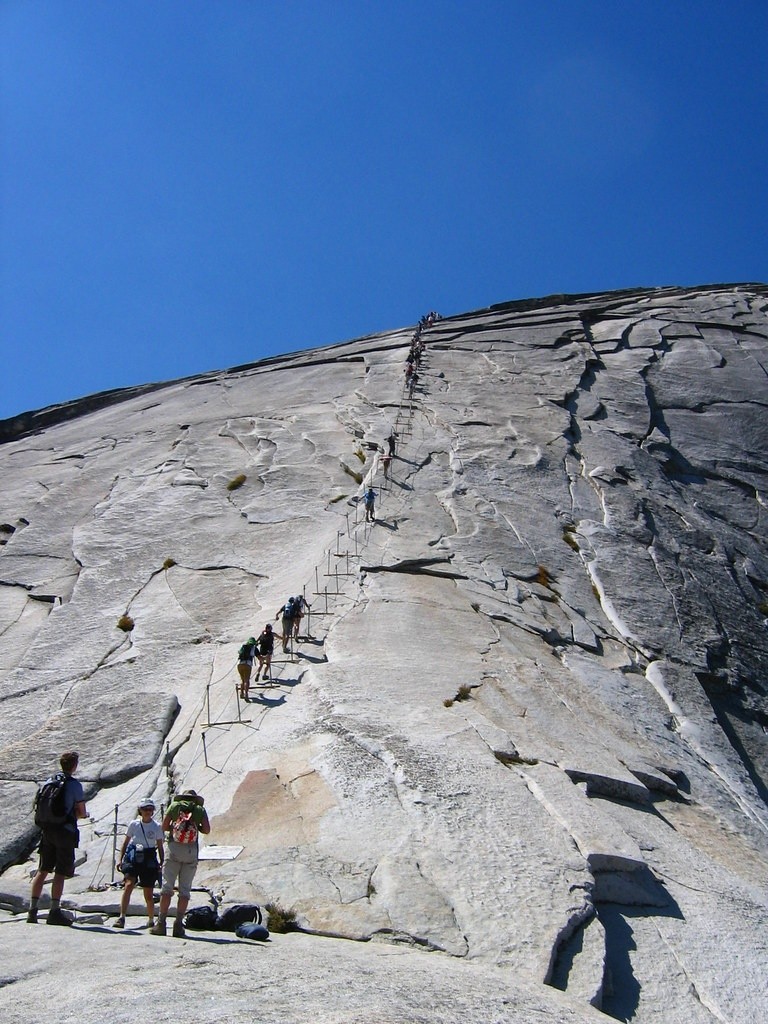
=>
[245,697,249,703]
[255,674,259,682]
[240,695,245,698]
[262,675,270,680]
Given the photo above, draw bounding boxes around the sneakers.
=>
[147,922,154,928]
[47,908,73,926]
[27,907,38,923]
[150,919,166,935]
[173,921,184,937]
[113,917,125,928]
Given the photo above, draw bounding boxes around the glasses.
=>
[143,808,153,812]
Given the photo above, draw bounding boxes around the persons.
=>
[112,796,165,928]
[150,788,210,938]
[359,489,379,522]
[382,457,390,475]
[255,623,285,681]
[26,751,87,925]
[387,435,400,457]
[276,595,310,655]
[403,311,436,398]
[237,637,262,703]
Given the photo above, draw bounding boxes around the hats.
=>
[174,790,204,807]
[139,798,154,808]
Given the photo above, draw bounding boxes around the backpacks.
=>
[239,644,253,659]
[215,904,262,932]
[173,801,197,843]
[35,775,75,830]
[294,596,301,606]
[285,602,294,618]
[186,906,218,929]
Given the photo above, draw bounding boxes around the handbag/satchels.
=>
[235,921,269,940]
